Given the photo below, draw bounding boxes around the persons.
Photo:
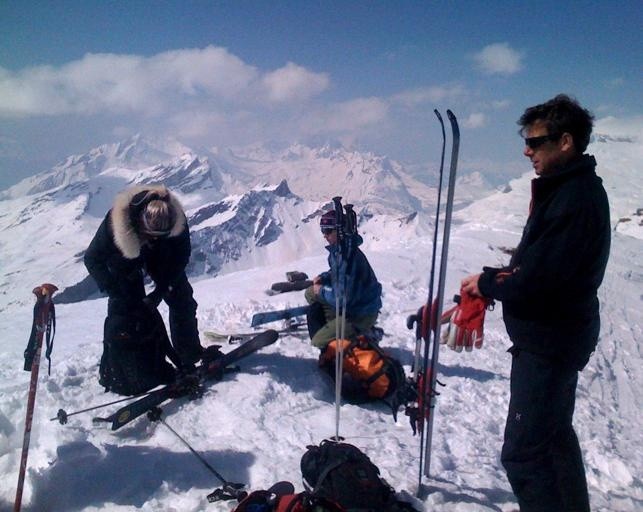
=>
[304,210,382,327]
[460,95,612,511]
[84,186,203,366]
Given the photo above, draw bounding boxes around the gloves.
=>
[440,285,485,352]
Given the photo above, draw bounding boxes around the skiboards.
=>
[387,107,461,498]
[203,305,310,339]
[112,328,278,431]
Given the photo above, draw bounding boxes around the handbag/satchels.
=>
[99,295,186,396]
[232,440,415,512]
[318,336,404,402]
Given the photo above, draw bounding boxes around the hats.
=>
[320,210,345,227]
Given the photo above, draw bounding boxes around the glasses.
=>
[321,227,334,234]
[524,134,551,148]
